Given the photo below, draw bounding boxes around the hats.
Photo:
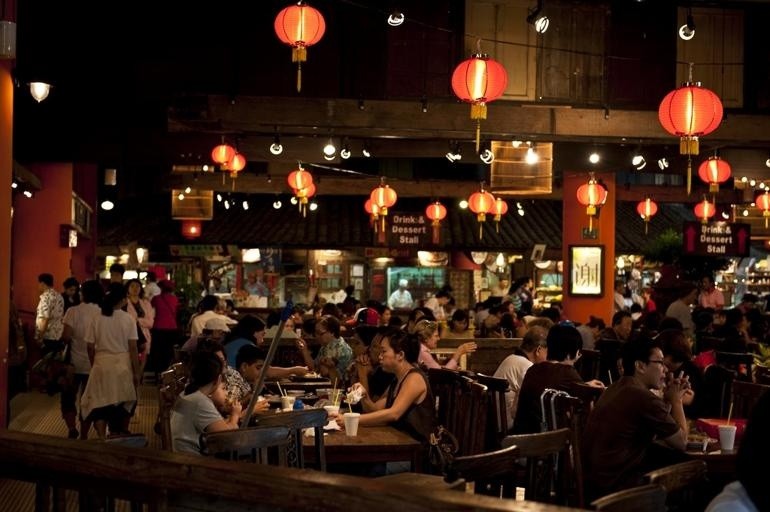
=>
[203,317,231,332]
[399,279,408,287]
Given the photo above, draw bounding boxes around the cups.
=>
[719,424,737,450]
[296,328,301,337]
[344,412,360,438]
[324,405,339,417]
[282,396,295,411]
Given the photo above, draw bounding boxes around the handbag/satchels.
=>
[28,336,73,398]
[528,388,577,503]
[426,424,458,467]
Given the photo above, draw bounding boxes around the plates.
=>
[287,390,306,394]
[685,435,718,448]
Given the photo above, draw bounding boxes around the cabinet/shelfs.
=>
[387,266,447,300]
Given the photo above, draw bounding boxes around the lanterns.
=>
[657,61,724,195]
[273,0,326,93]
[693,194,716,225]
[698,146,731,195]
[576,174,607,233]
[451,38,508,152]
[362,175,508,243]
[754,190,770,229]
[286,161,317,218]
[209,135,247,191]
[635,197,658,235]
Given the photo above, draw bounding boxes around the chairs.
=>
[159,327,770,512]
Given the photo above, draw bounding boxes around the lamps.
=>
[525,141,541,166]
[677,5,695,41]
[631,145,648,171]
[387,8,406,26]
[216,191,250,212]
[657,146,673,170]
[524,6,550,34]
[269,124,373,162]
[14,63,53,104]
[445,140,462,162]
[721,203,736,219]
[517,198,534,217]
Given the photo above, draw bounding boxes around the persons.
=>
[170,272,769,511]
[34,264,182,441]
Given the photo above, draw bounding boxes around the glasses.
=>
[315,330,326,337]
[579,353,582,359]
[424,320,430,330]
[648,358,666,367]
